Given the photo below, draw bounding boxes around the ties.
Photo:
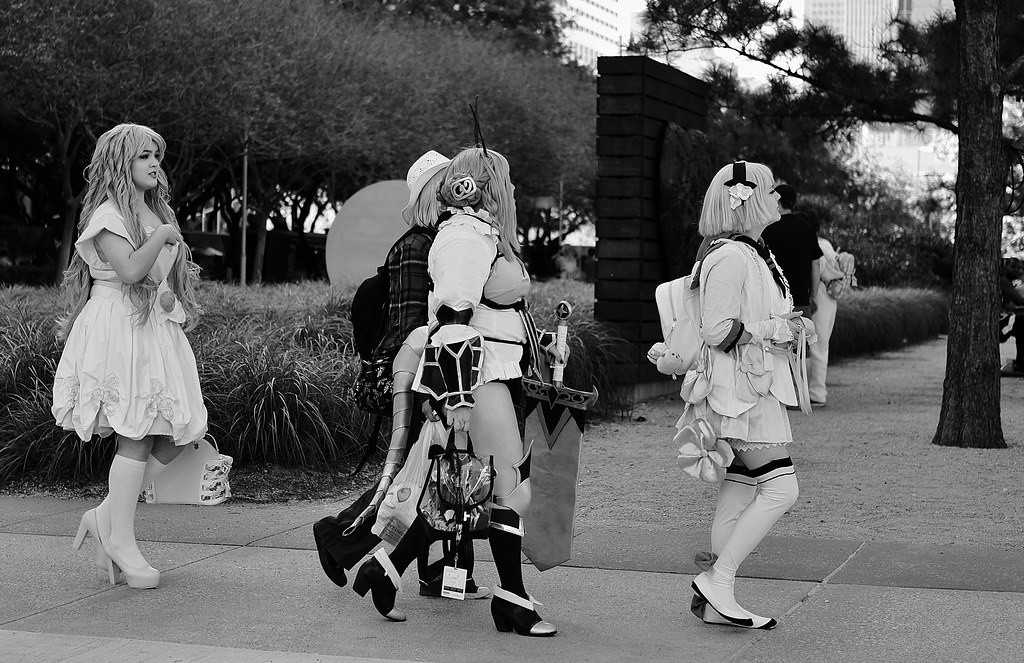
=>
[736,234,788,301]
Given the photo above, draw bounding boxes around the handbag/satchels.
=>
[144,433,234,505]
[416,425,497,545]
[353,330,399,416]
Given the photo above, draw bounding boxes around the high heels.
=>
[490,584,558,636]
[352,546,415,621]
[73,508,123,574]
[106,538,160,589]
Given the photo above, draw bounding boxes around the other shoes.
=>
[690,552,755,626]
[314,516,349,587]
[420,574,489,598]
[811,399,824,406]
[690,594,777,630]
[1012,359,1024,371]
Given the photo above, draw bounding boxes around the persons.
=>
[690,158,803,629]
[50,122,209,591]
[760,174,844,409]
[311,147,569,638]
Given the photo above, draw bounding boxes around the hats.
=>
[401,150,451,227]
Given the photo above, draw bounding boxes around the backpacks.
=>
[828,251,855,301]
[351,226,436,352]
[647,238,751,379]
